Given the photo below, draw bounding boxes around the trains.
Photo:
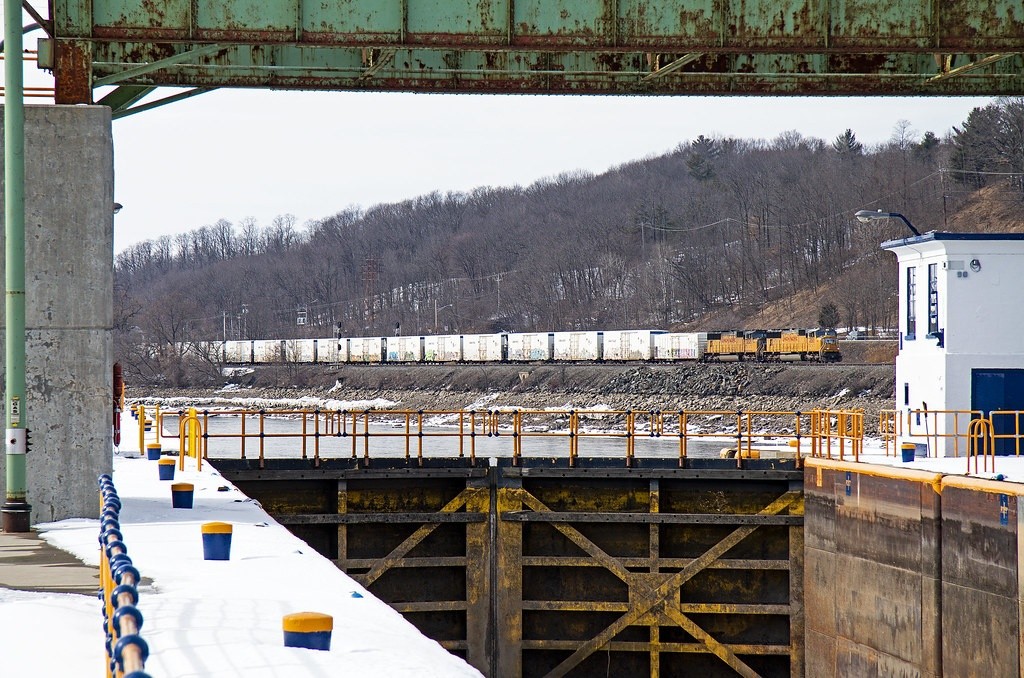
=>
[134,325,843,364]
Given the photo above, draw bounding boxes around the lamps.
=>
[855,210,921,237]
